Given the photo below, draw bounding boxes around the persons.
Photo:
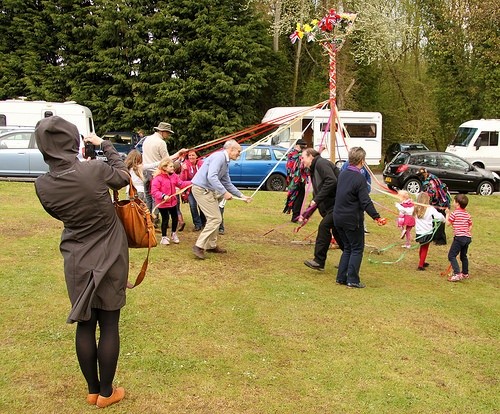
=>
[416,168,453,246]
[299,148,340,272]
[332,146,388,288]
[415,192,444,271]
[445,194,473,281]
[283,140,325,225]
[395,189,416,249]
[123,121,253,259]
[35,115,131,409]
[341,147,372,235]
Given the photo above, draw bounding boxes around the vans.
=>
[444,118,500,172]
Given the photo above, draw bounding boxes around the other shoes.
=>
[219,227,224,235]
[206,246,227,253]
[86,386,125,407]
[171,232,180,243]
[417,263,429,271]
[448,272,469,282]
[193,245,204,259]
[160,236,170,245]
[336,279,367,289]
[304,260,324,271]
[195,226,201,231]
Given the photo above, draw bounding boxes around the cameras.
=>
[84,141,96,159]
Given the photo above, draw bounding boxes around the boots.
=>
[176,214,185,232]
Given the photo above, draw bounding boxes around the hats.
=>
[154,122,174,134]
[296,139,307,145]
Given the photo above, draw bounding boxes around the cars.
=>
[228,144,294,192]
[0,125,51,183]
[384,142,430,168]
[382,149,500,196]
[99,132,132,144]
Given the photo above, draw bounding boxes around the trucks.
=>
[0,97,96,137]
[256,106,382,170]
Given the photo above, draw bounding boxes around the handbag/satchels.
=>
[112,169,158,248]
